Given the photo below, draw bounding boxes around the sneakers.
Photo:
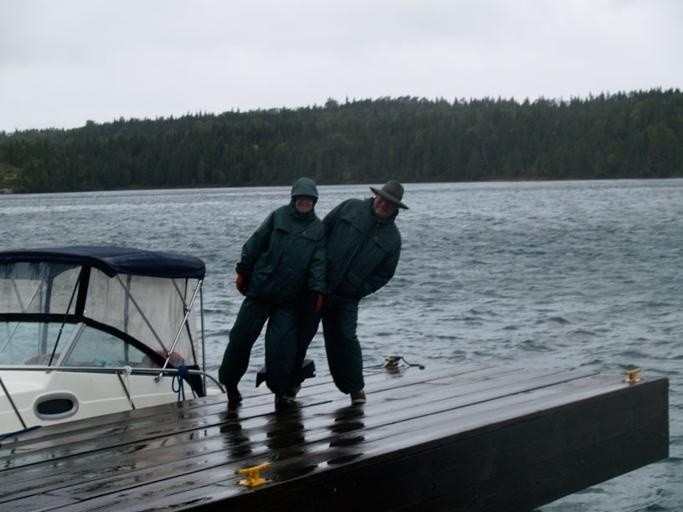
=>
[228,390,242,410]
[282,384,301,400]
[350,390,366,404]
[274,396,298,412]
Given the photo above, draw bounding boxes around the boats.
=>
[0,244,227,440]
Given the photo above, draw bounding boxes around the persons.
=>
[216,176,330,411]
[269,180,412,406]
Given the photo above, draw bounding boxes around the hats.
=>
[370,181,409,209]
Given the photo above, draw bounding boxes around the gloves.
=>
[314,293,323,312]
[237,273,247,295]
